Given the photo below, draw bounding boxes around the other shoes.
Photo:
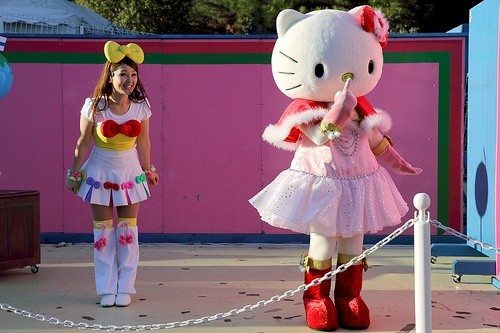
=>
[100,294,116,306]
[116,293,131,306]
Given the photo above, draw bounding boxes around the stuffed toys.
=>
[260,4,423,330]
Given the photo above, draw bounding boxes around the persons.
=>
[64,42,159,307]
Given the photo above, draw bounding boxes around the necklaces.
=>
[332,125,359,156]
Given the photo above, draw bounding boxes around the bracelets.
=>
[142,165,156,175]
[66,169,82,181]
